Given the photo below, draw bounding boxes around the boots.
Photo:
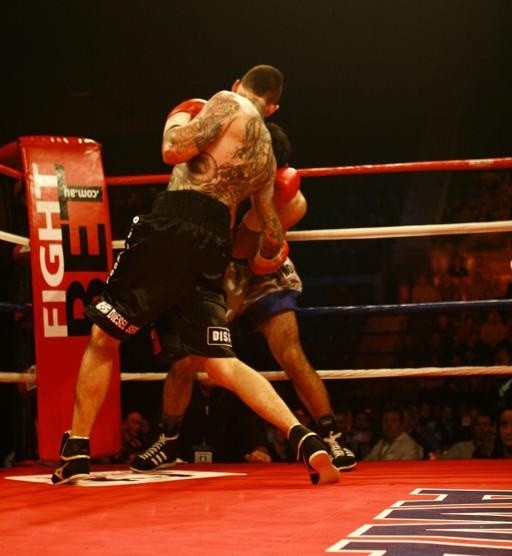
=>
[51,430,91,485]
[288,424,340,484]
[128,433,178,473]
[319,415,357,471]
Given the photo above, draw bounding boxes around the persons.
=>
[402,245,486,360]
[183,395,512,464]
[54,65,355,484]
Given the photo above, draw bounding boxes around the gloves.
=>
[162,98,206,134]
[244,166,300,234]
[247,240,289,277]
